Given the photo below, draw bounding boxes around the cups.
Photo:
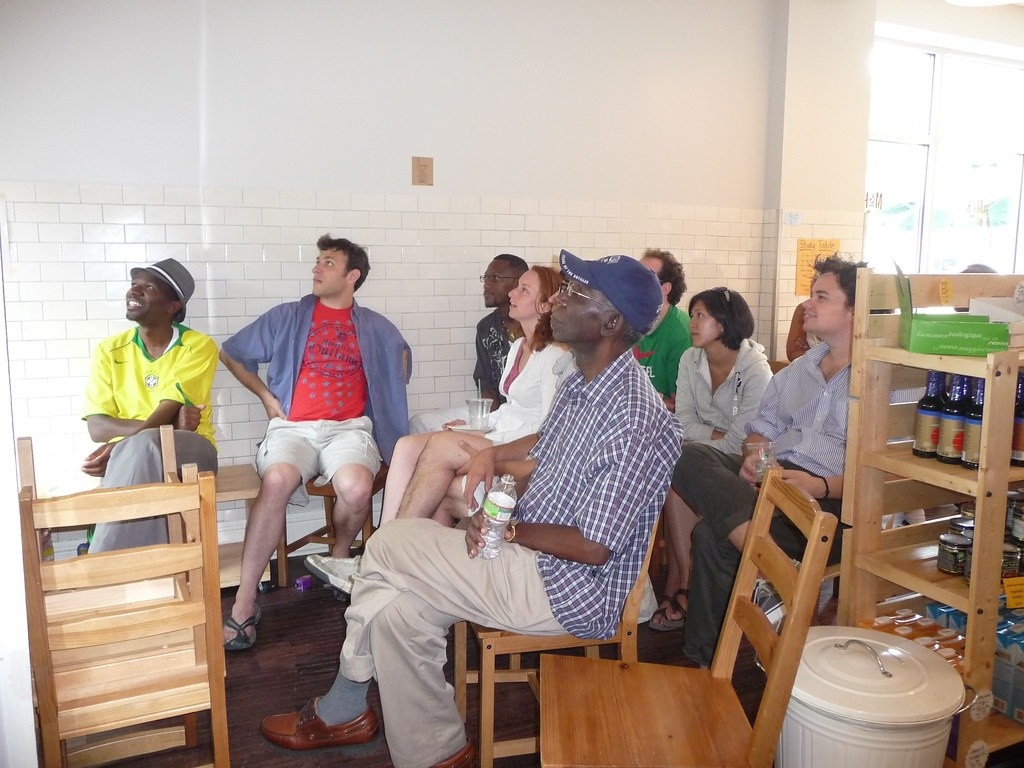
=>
[746,441,778,482]
[466,399,493,428]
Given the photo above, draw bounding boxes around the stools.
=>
[208,463,271,589]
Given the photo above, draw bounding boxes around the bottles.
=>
[1009,374,1024,468]
[475,474,516,561]
[911,370,985,471]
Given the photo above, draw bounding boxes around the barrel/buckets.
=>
[754,625,978,768]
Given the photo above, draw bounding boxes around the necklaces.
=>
[501,318,522,339]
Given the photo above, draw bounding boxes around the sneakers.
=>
[305,554,359,594]
[752,560,803,626]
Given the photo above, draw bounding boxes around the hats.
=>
[560,248,663,335]
[131,258,200,324]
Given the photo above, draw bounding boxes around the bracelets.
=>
[506,525,516,542]
[816,475,830,498]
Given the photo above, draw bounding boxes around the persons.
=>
[670,254,894,680]
[260,245,683,767]
[648,285,773,630]
[302,266,576,598]
[220,232,411,652]
[634,248,691,416]
[786,273,830,360]
[80,258,220,556]
[472,254,528,412]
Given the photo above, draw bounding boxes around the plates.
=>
[447,425,494,434]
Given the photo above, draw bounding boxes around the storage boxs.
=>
[925,595,1023,723]
[895,264,1023,357]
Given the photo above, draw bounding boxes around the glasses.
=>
[711,287,730,311]
[559,280,619,315]
[480,273,521,283]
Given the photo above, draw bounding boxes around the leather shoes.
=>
[260,697,381,757]
[418,736,476,768]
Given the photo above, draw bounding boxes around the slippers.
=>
[649,589,689,632]
[224,602,261,650]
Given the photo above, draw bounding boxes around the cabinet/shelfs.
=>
[838,267,1024,768]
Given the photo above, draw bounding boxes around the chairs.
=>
[16,415,838,768]
